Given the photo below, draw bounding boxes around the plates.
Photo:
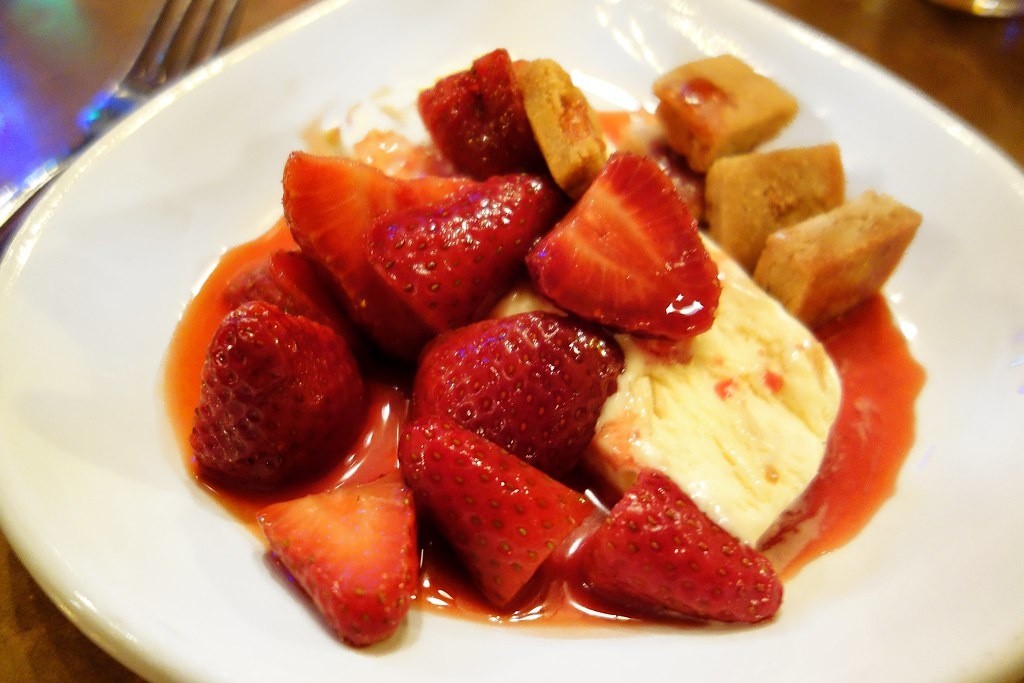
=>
[0,0,1024,683]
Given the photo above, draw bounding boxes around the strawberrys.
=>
[186,47,783,651]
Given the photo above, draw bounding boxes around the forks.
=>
[0,0,244,228]
